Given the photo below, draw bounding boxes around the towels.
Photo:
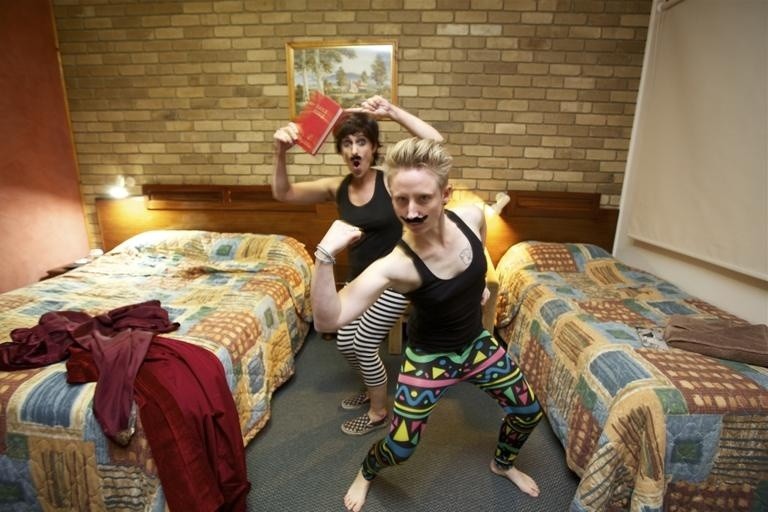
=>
[663,310,767,368]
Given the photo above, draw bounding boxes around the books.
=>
[292,91,346,155]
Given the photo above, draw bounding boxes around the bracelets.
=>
[311,244,336,268]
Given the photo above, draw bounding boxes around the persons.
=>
[300,135,544,511]
[268,95,449,436]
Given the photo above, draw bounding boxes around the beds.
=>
[482,189,767,511]
[0,184,350,511]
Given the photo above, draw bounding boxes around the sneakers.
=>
[342,391,369,409]
[341,411,389,435]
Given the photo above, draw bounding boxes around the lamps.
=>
[108,174,137,199]
[485,192,509,219]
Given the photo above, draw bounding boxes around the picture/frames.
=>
[283,35,398,122]
